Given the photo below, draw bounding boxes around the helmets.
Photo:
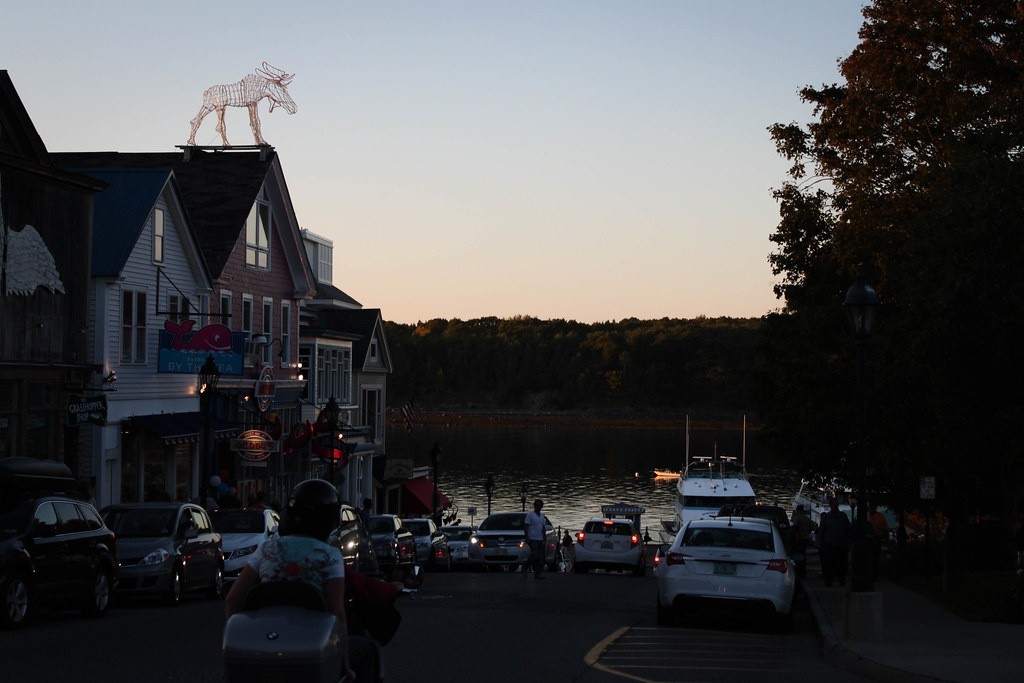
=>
[278,479,340,540]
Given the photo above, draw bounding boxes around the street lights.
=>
[430,444,441,518]
[198,356,220,501]
[848,491,857,525]
[520,485,528,510]
[485,479,496,514]
[326,395,339,486]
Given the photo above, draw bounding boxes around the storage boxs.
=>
[220,605,347,683]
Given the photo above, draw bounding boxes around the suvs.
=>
[1,455,119,628]
[97,500,228,602]
[365,514,415,570]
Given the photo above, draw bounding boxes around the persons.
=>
[74,480,96,514]
[225,478,405,682]
[561,530,573,560]
[146,480,186,502]
[792,498,889,587]
[190,487,270,512]
[523,499,547,579]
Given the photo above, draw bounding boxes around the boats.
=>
[674,454,757,528]
[793,473,899,532]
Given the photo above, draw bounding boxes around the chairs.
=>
[419,526,425,530]
[748,537,766,549]
[461,532,469,538]
[594,524,603,533]
[376,521,392,531]
[694,531,713,544]
[617,525,630,535]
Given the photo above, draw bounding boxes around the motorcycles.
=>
[220,563,421,683]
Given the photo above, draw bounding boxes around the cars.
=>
[652,544,673,577]
[400,517,448,571]
[573,518,646,577]
[330,503,376,572]
[654,514,798,631]
[207,506,279,586]
[477,513,560,571]
[439,526,479,564]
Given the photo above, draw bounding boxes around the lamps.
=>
[102,370,116,383]
[252,332,285,358]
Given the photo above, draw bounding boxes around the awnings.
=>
[131,411,239,445]
[403,478,452,515]
